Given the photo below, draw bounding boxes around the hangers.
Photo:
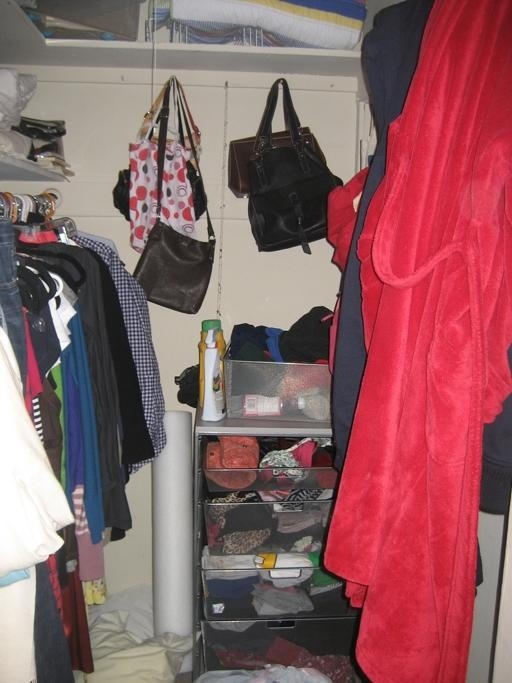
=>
[0,193,86,312]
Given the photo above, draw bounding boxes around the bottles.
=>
[198,319,228,408]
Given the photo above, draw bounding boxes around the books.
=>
[9,114,76,179]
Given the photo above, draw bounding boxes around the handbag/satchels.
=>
[228,78,326,198]
[112,78,207,253]
[248,79,343,254]
[133,221,216,314]
